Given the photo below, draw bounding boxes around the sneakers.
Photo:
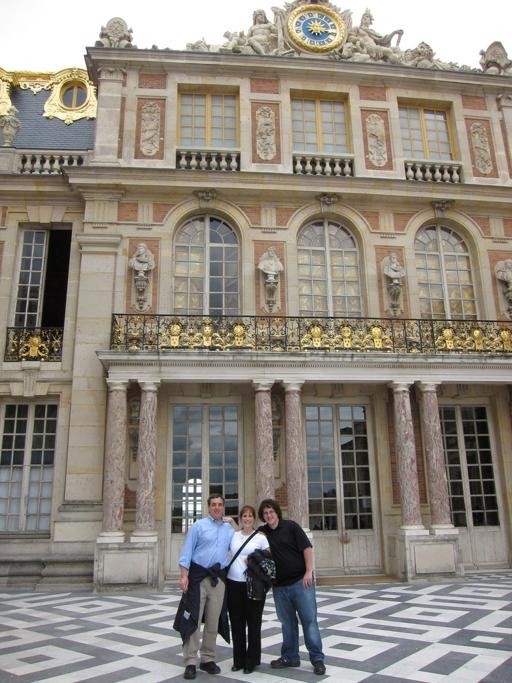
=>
[313,659,326,675]
[184,664,196,679]
[200,661,221,674]
[232,664,255,674]
[271,656,300,668]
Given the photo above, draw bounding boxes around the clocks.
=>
[287,3,345,52]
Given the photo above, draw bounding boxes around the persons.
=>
[241,8,282,55]
[225,505,276,673]
[354,6,404,60]
[172,493,238,679]
[224,498,326,675]
